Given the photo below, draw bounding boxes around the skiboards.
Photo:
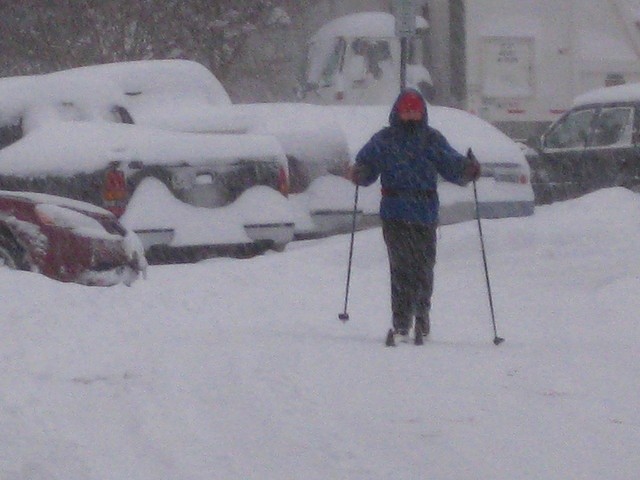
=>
[385,329,425,346]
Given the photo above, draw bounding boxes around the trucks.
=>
[297,1,640,144]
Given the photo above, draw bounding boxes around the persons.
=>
[351,87,481,343]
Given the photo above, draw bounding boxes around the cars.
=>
[367,104,534,227]
[0,126,294,263]
[1,187,148,288]
[516,82,640,205]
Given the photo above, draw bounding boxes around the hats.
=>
[399,93,424,113]
